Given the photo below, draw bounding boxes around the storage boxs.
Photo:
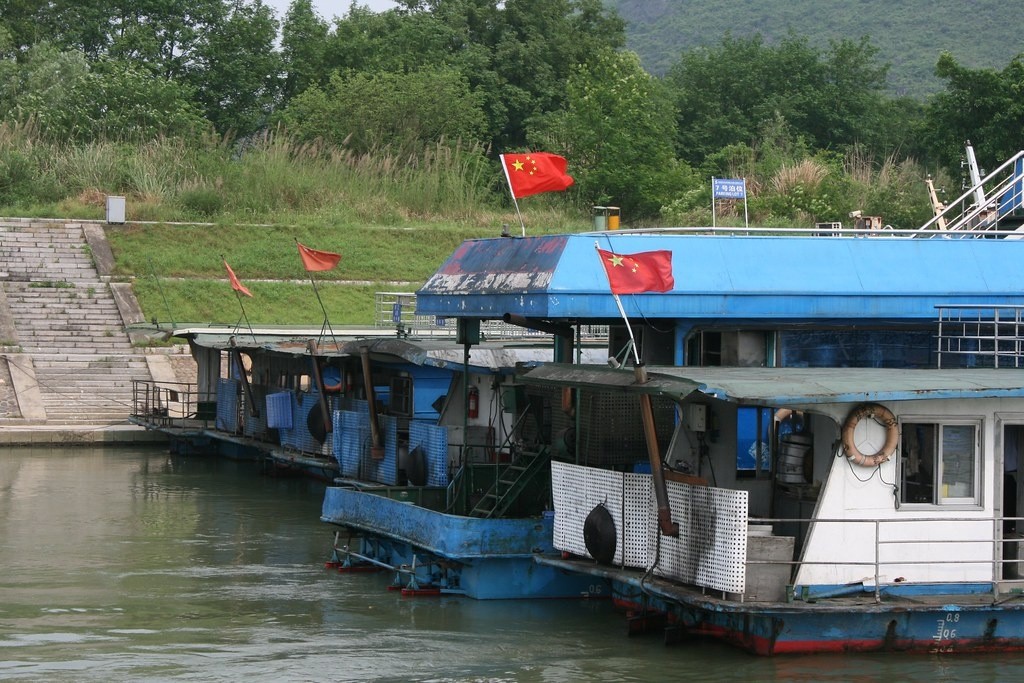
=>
[266,390,293,431]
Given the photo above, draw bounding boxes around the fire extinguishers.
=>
[468,385,479,418]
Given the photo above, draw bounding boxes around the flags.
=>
[599,248,674,293]
[224,261,253,297]
[299,244,341,272]
[502,152,574,198]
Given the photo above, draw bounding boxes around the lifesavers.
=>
[841,403,899,467]
[767,408,803,440]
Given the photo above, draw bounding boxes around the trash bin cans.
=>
[592,206,607,231]
[105,195,126,225]
[606,207,621,230]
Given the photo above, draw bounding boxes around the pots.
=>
[583,503,616,566]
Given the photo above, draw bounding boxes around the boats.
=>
[129,225,1024,655]
